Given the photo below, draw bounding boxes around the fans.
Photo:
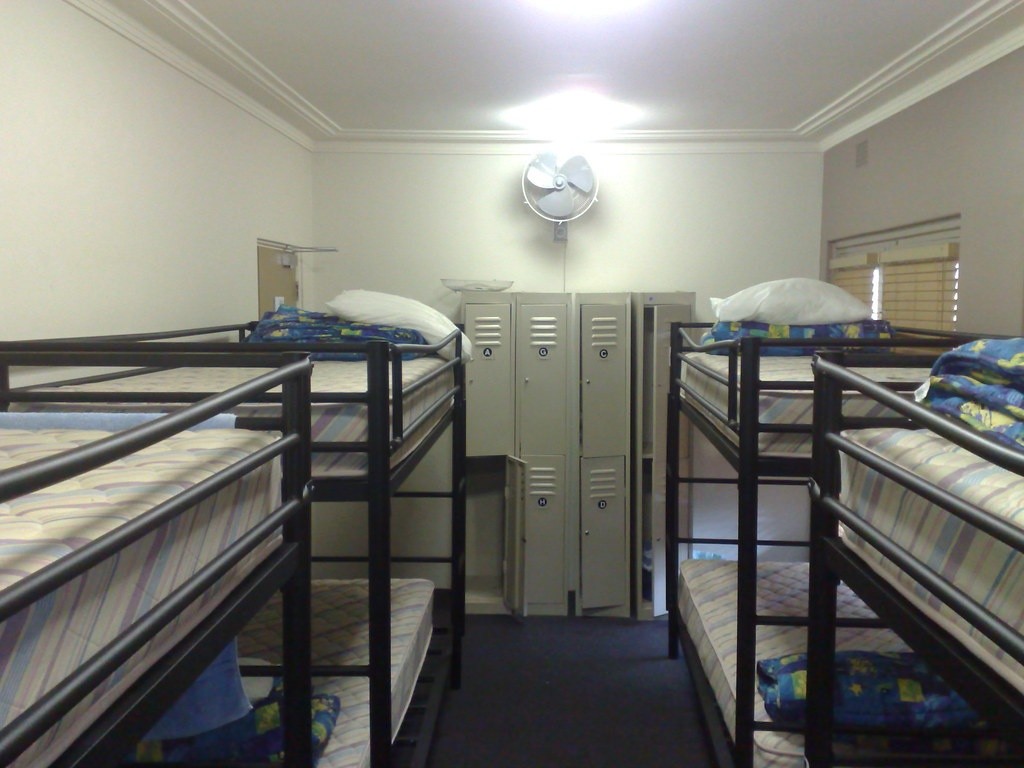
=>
[520,139,602,224]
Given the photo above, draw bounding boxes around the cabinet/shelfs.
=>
[463,289,696,623]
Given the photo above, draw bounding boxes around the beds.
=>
[0,291,474,768]
[663,276,1024,768]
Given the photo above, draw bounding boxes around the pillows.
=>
[710,276,873,326]
[326,289,472,365]
[235,656,282,705]
